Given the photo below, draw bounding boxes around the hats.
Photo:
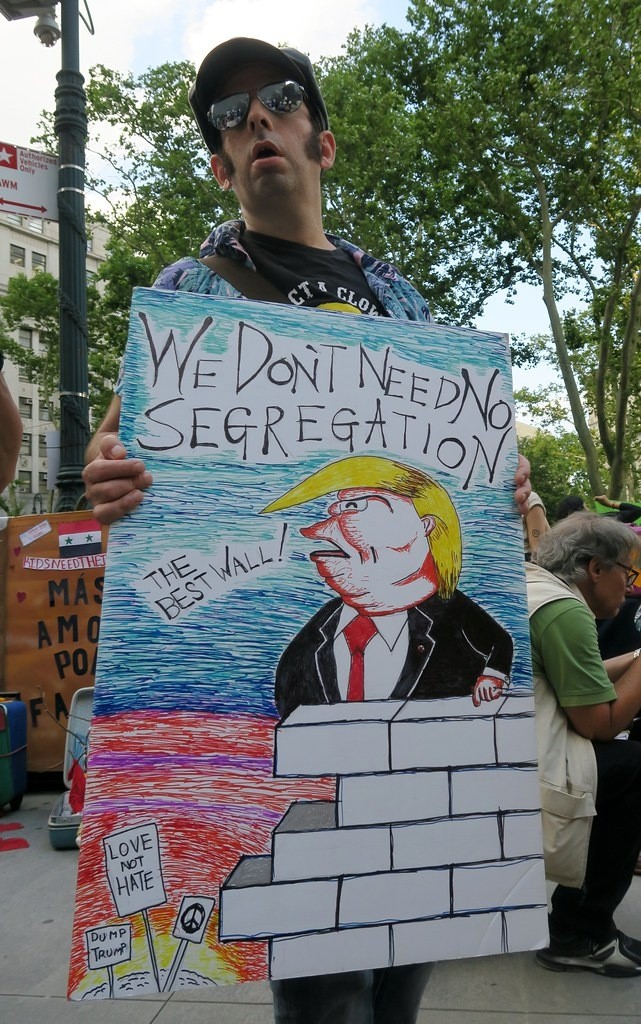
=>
[188,37,330,154]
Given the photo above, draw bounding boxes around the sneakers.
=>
[536,912,641,978]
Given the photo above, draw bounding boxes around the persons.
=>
[521,491,552,563]
[0,373,22,496]
[558,494,641,524]
[83,36,533,1024]
[522,509,641,977]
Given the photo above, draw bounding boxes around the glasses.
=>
[608,558,640,587]
[206,79,324,134]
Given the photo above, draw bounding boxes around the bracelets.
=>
[634,647,641,658]
[613,502,616,508]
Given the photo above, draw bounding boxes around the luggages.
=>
[0,690,28,811]
[48,687,96,848]
